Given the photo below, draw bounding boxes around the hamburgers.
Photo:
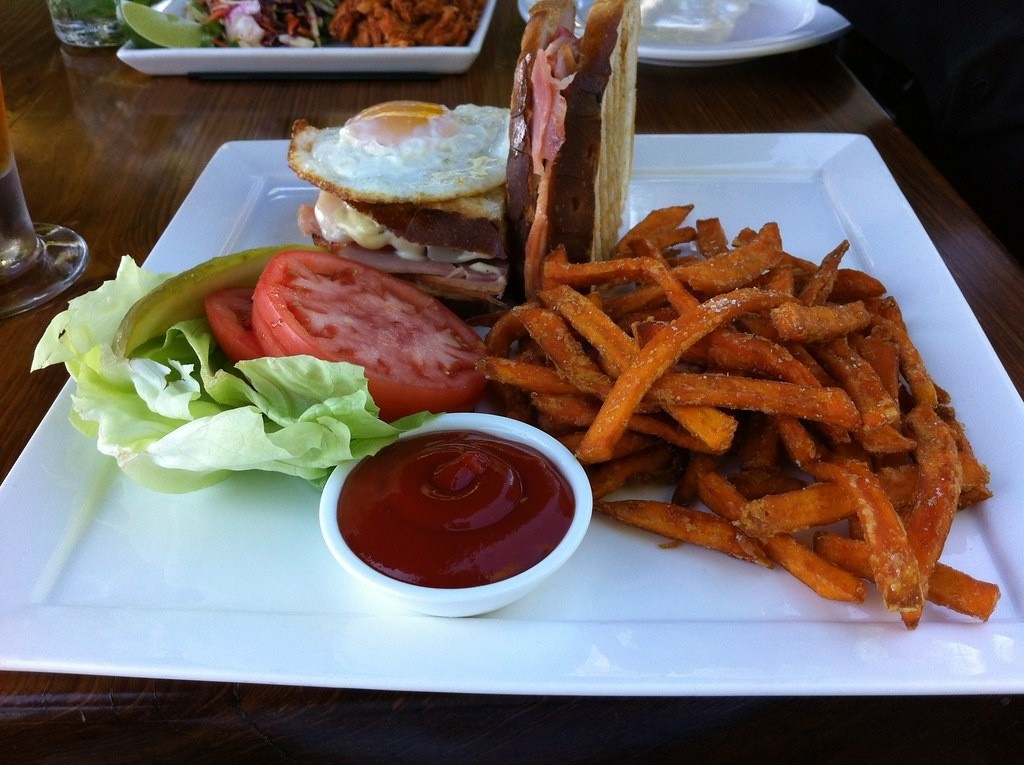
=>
[502,0,640,291]
[286,95,510,304]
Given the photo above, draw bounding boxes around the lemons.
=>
[113,1,202,49]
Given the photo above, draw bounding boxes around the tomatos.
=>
[207,251,487,417]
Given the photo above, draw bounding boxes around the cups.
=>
[43,0,148,49]
[1,77,90,318]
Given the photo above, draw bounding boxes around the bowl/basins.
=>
[319,412,594,616]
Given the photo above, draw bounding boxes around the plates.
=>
[116,1,497,74]
[515,1,851,67]
[1,132,1024,697]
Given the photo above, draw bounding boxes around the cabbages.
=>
[24,251,444,495]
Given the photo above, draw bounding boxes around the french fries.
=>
[480,204,999,626]
[327,0,477,47]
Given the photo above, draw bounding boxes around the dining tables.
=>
[0,0,1024,765]
